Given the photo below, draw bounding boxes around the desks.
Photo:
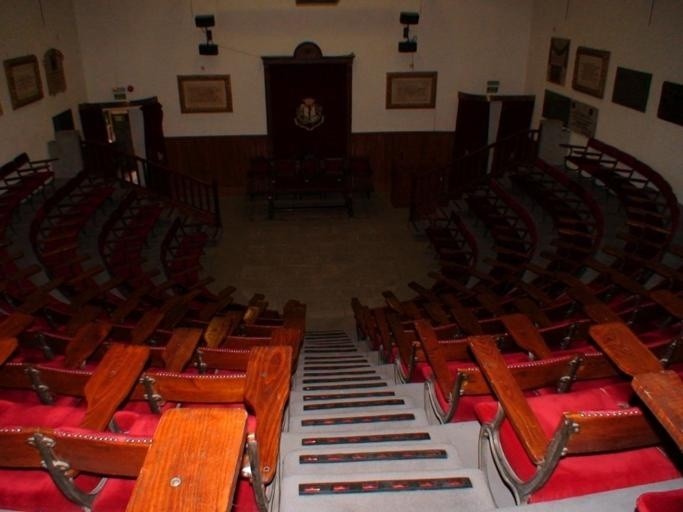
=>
[264,175,374,217]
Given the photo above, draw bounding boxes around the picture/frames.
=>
[386,72,437,109]
[3,55,44,110]
[177,75,232,113]
[572,47,609,98]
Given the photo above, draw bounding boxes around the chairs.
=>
[0,153,305,511]
[352,140,683,512]
